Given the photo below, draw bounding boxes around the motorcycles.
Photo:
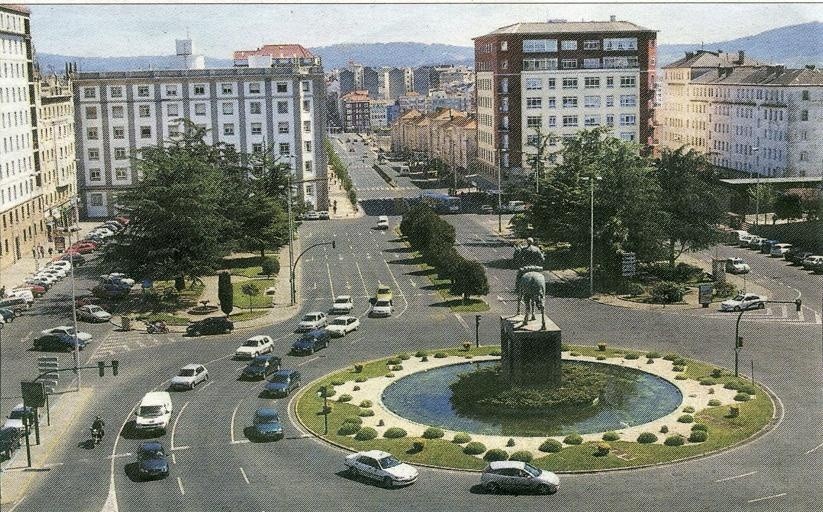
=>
[90,426,104,446]
[144,320,170,334]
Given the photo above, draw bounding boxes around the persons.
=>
[91,416,105,439]
[161,320,167,332]
[328,198,338,214]
[513,237,546,294]
[0,286,6,298]
[328,169,342,190]
[32,232,53,259]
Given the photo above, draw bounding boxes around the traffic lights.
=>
[319,386,326,398]
[739,337,743,347]
[98,367,104,376]
[477,314,482,326]
[112,365,118,377]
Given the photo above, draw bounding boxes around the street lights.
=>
[492,148,508,232]
[580,176,604,294]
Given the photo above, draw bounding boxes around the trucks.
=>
[421,191,461,214]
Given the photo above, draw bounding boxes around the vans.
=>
[479,460,562,495]
[135,391,173,436]
[378,216,389,230]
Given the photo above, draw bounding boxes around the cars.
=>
[721,293,765,313]
[372,299,394,317]
[34,334,85,352]
[326,316,359,338]
[375,286,392,300]
[3,404,38,436]
[254,407,284,442]
[235,335,275,358]
[1,218,134,325]
[296,312,328,331]
[291,329,331,355]
[171,363,208,391]
[186,317,233,335]
[343,450,419,488]
[40,325,93,344]
[264,370,301,397]
[243,355,281,380]
[136,441,169,479]
[725,226,822,274]
[297,210,330,220]
[333,296,352,314]
